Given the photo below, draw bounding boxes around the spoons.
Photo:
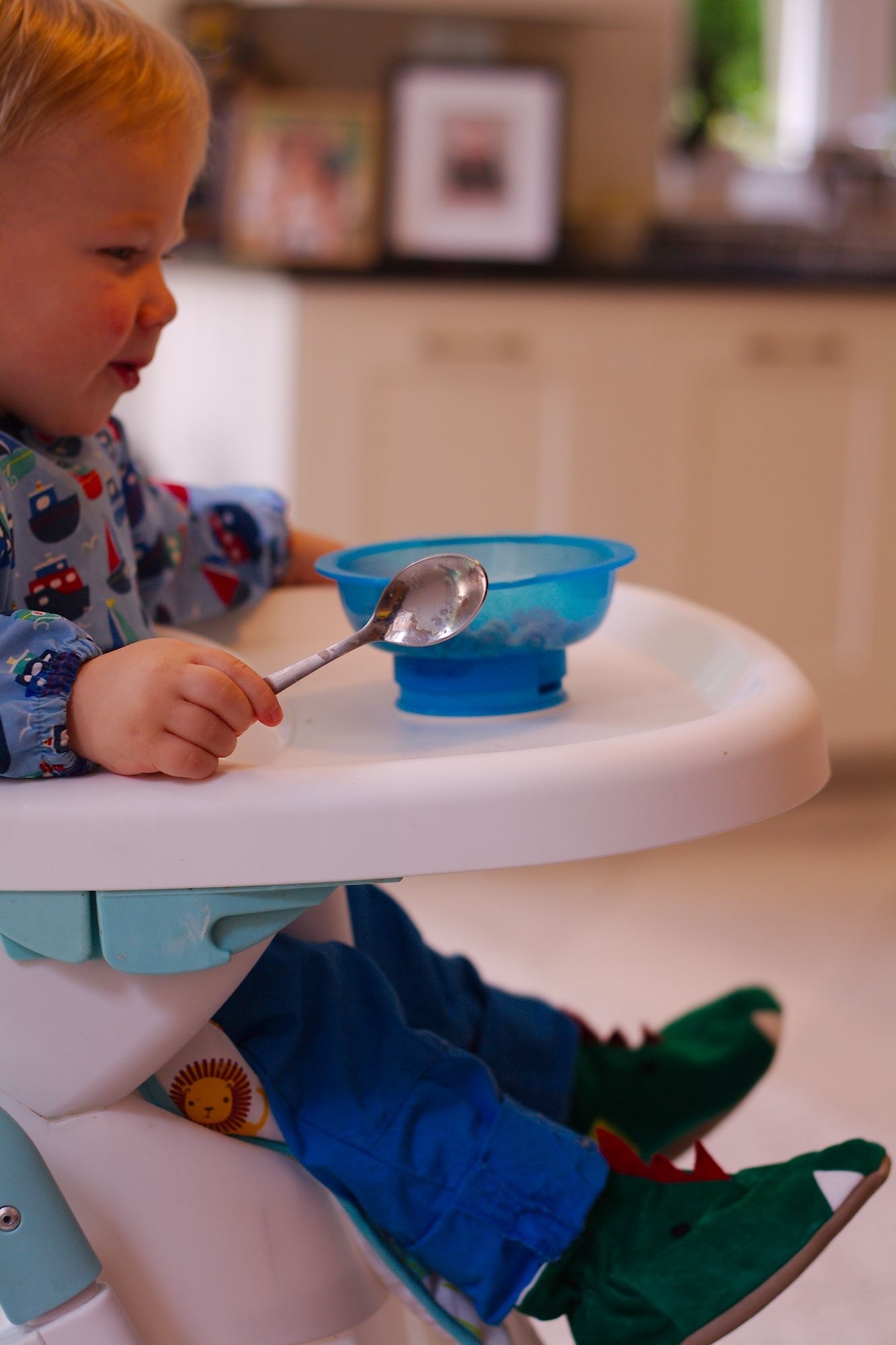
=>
[262,553,489,694]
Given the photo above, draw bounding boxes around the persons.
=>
[0,0,891,1345]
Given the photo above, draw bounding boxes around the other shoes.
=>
[512,1122,891,1345]
[577,983,781,1159]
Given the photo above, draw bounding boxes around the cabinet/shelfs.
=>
[103,227,896,793]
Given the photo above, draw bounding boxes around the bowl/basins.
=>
[313,534,639,718]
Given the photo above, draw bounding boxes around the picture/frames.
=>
[211,75,389,273]
[386,55,575,275]
